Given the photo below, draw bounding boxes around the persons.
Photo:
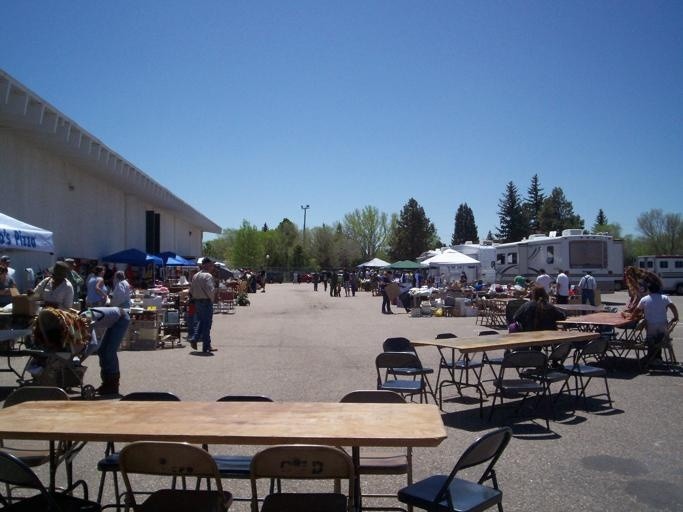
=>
[78,306,130,394]
[505,290,529,354]
[25,261,74,368]
[186,258,217,356]
[459,271,467,288]
[1,255,16,350]
[512,287,566,370]
[629,276,678,364]
[312,268,449,314]
[512,268,571,304]
[38,257,131,311]
[578,271,597,316]
[236,266,267,293]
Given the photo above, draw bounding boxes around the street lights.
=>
[299,201,312,235]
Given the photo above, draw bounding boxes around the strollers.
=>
[4,304,107,403]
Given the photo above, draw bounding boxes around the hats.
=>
[202,258,215,265]
[1,256,11,262]
[49,258,76,276]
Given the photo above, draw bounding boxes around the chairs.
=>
[196,395,281,502]
[1,450,103,512]
[397,427,511,512]
[333,390,413,512]
[249,444,354,512]
[374,329,618,429]
[1,384,84,495]
[474,298,508,330]
[610,316,678,371]
[118,443,233,512]
[97,392,187,512]
[0,313,43,384]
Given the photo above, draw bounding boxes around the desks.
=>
[566,311,644,367]
[493,296,530,303]
[551,304,607,316]
[1,400,447,512]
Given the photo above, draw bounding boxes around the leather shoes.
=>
[189,338,217,356]
